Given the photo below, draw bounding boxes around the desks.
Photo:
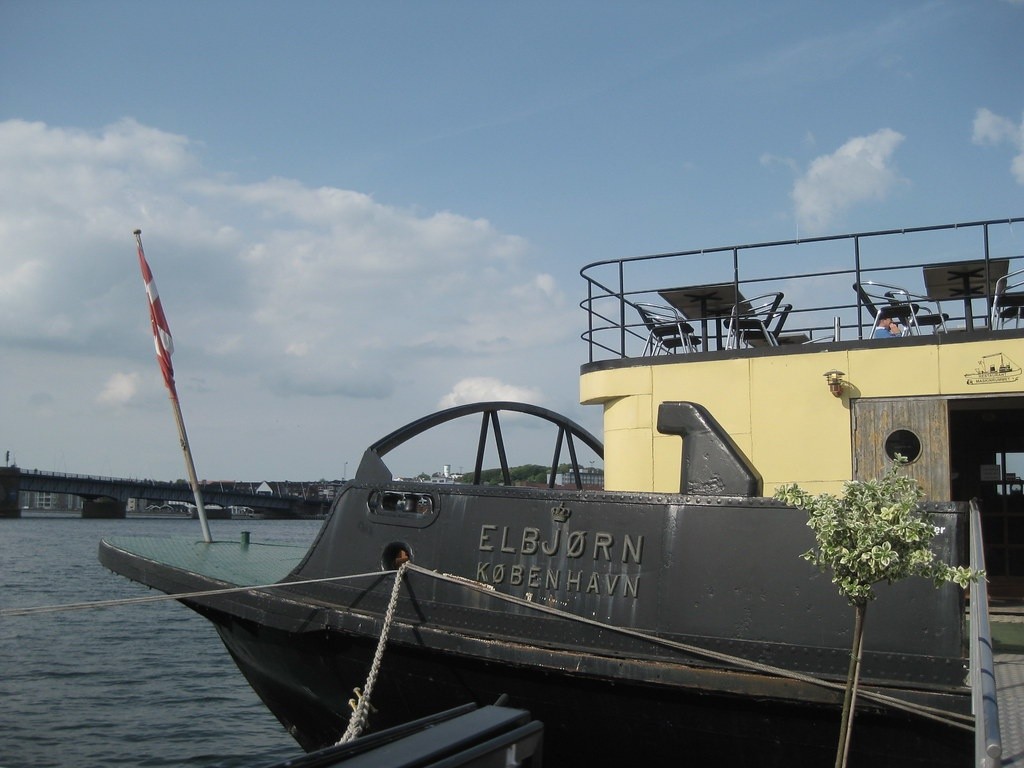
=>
[657,282,756,353]
[923,257,1010,331]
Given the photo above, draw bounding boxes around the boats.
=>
[97,218,1024,768]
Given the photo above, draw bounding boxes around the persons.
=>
[874,317,902,338]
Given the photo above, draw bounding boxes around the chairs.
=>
[988,267,1024,330]
[852,281,951,340]
[723,291,794,351]
[634,301,702,355]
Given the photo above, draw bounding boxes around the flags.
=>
[136,242,176,396]
